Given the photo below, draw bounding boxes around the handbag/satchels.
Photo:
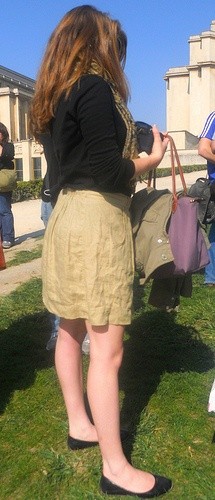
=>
[0,159,17,193]
[147,135,211,276]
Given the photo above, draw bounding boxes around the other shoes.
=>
[101,472,172,497]
[67,429,127,449]
[2,241,15,249]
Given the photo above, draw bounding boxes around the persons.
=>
[198,110,215,286]
[29,5,173,497]
[0,122,15,248]
[39,172,90,354]
